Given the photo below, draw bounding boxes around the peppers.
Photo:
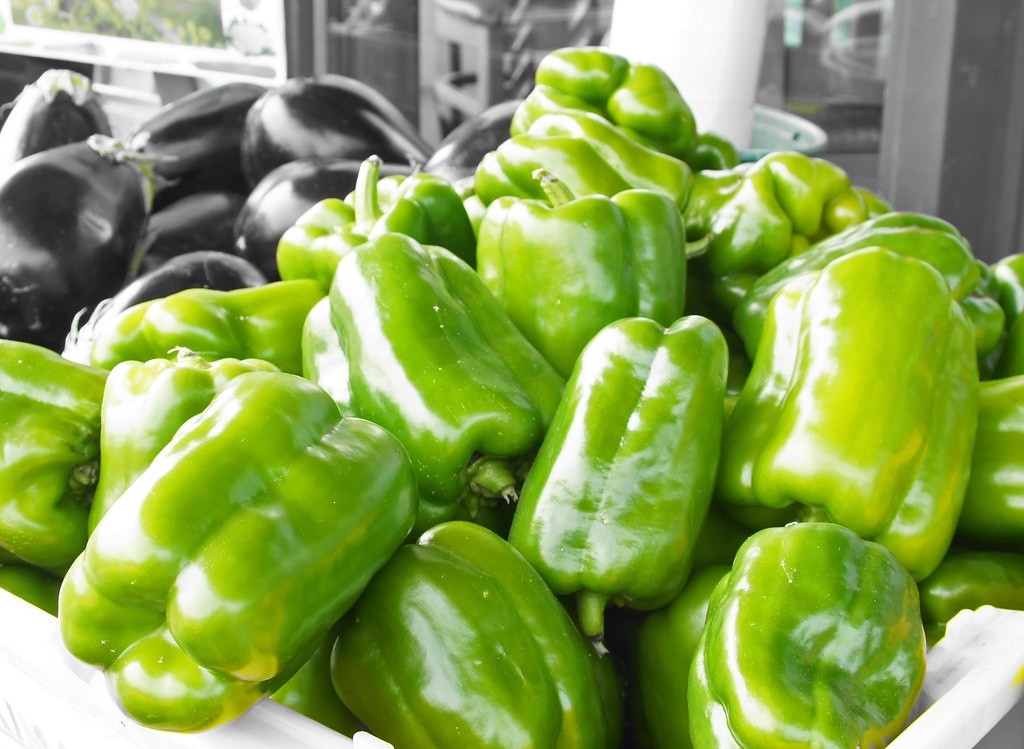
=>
[2,47,1024,747]
